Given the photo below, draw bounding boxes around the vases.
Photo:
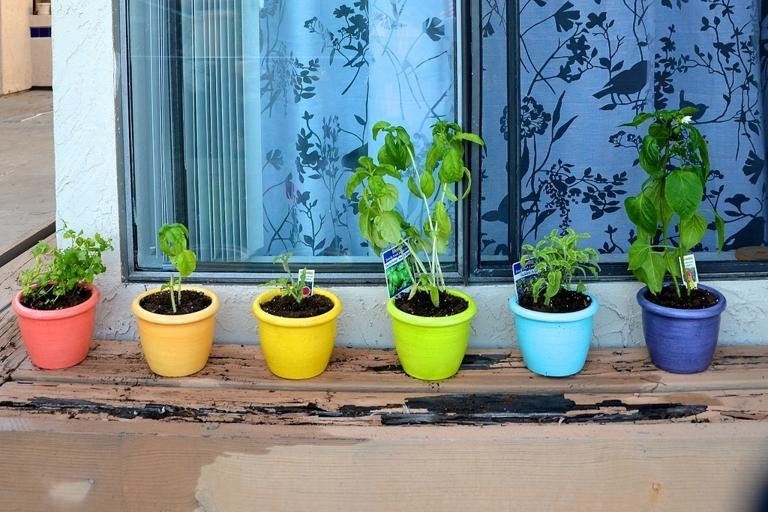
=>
[635,282,727,374]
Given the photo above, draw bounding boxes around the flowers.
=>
[614,105,726,298]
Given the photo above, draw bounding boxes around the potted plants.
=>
[130,222,221,379]
[252,251,344,382]
[508,227,601,379]
[11,210,116,370]
[342,120,484,382]
